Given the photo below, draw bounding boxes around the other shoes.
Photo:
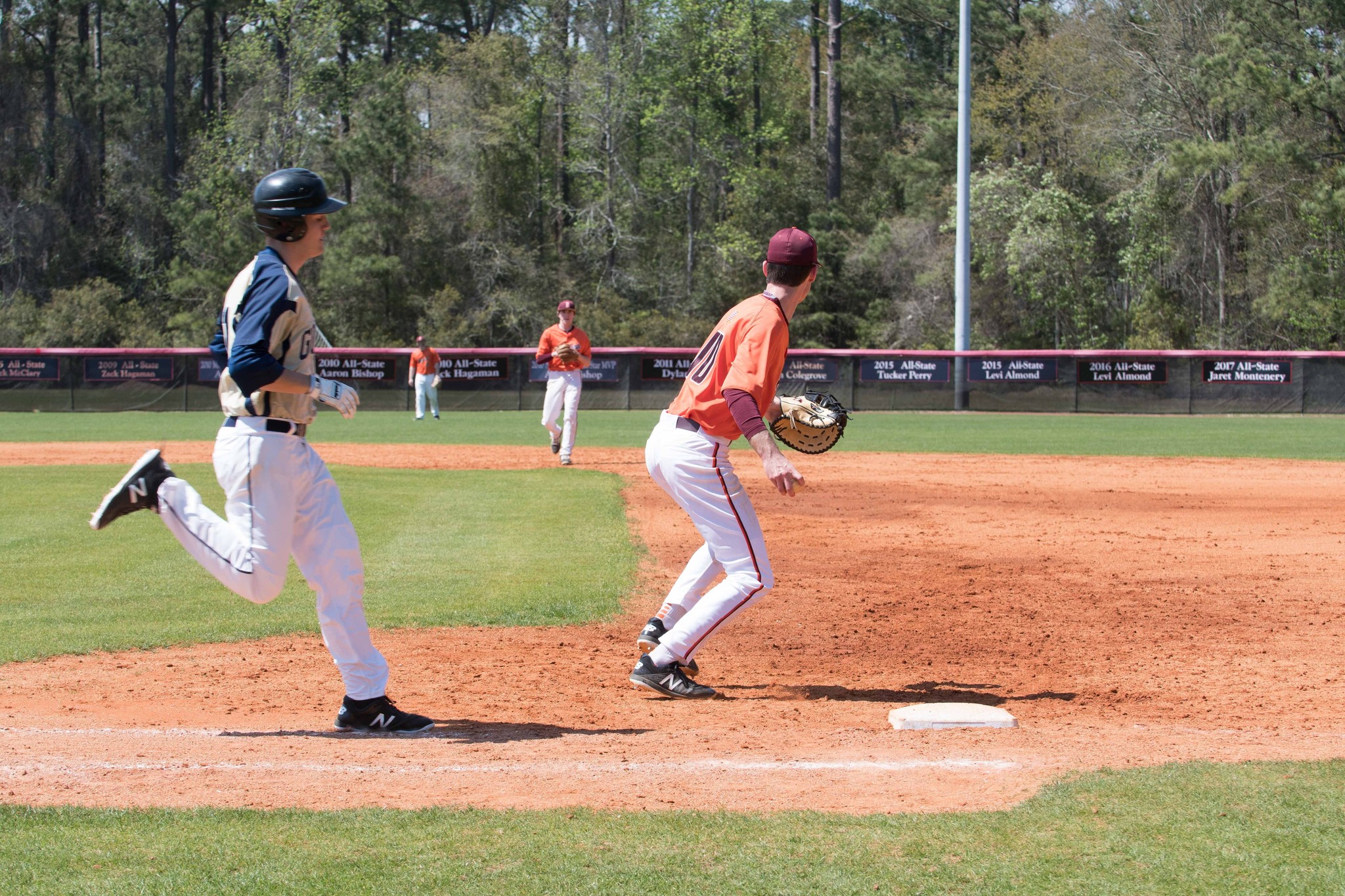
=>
[434,416,439,420]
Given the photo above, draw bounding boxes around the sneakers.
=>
[630,652,717,700]
[551,426,562,453]
[635,617,699,677]
[334,696,434,733]
[560,454,572,464]
[88,449,173,530]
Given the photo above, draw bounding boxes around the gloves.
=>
[307,374,359,418]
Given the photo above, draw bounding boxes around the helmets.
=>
[254,169,349,242]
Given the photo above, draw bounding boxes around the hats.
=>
[558,300,576,312]
[767,227,822,268]
[416,336,425,342]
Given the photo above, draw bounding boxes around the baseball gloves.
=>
[431,375,442,388]
[768,389,854,456]
[554,343,579,365]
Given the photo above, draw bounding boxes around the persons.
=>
[535,300,592,468]
[89,168,435,735]
[630,227,827,701]
[409,336,443,421]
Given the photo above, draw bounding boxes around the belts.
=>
[223,416,306,437]
[676,417,732,447]
